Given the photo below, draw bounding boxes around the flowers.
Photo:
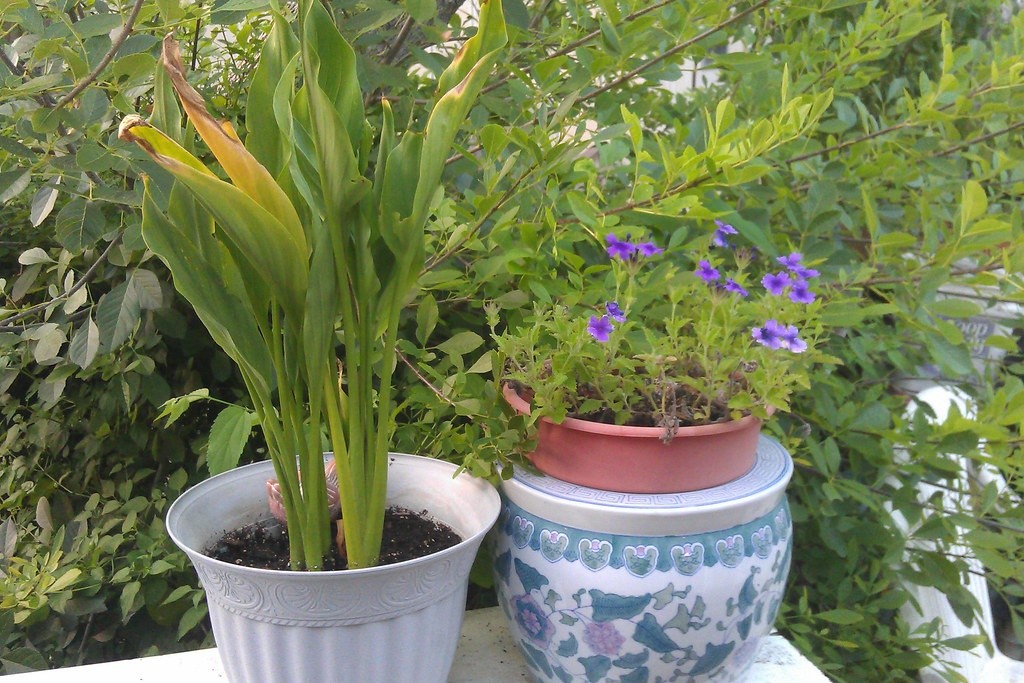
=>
[453,221,843,490]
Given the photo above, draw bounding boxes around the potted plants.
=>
[117,0,503,683]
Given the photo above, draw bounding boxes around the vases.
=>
[488,361,793,683]
